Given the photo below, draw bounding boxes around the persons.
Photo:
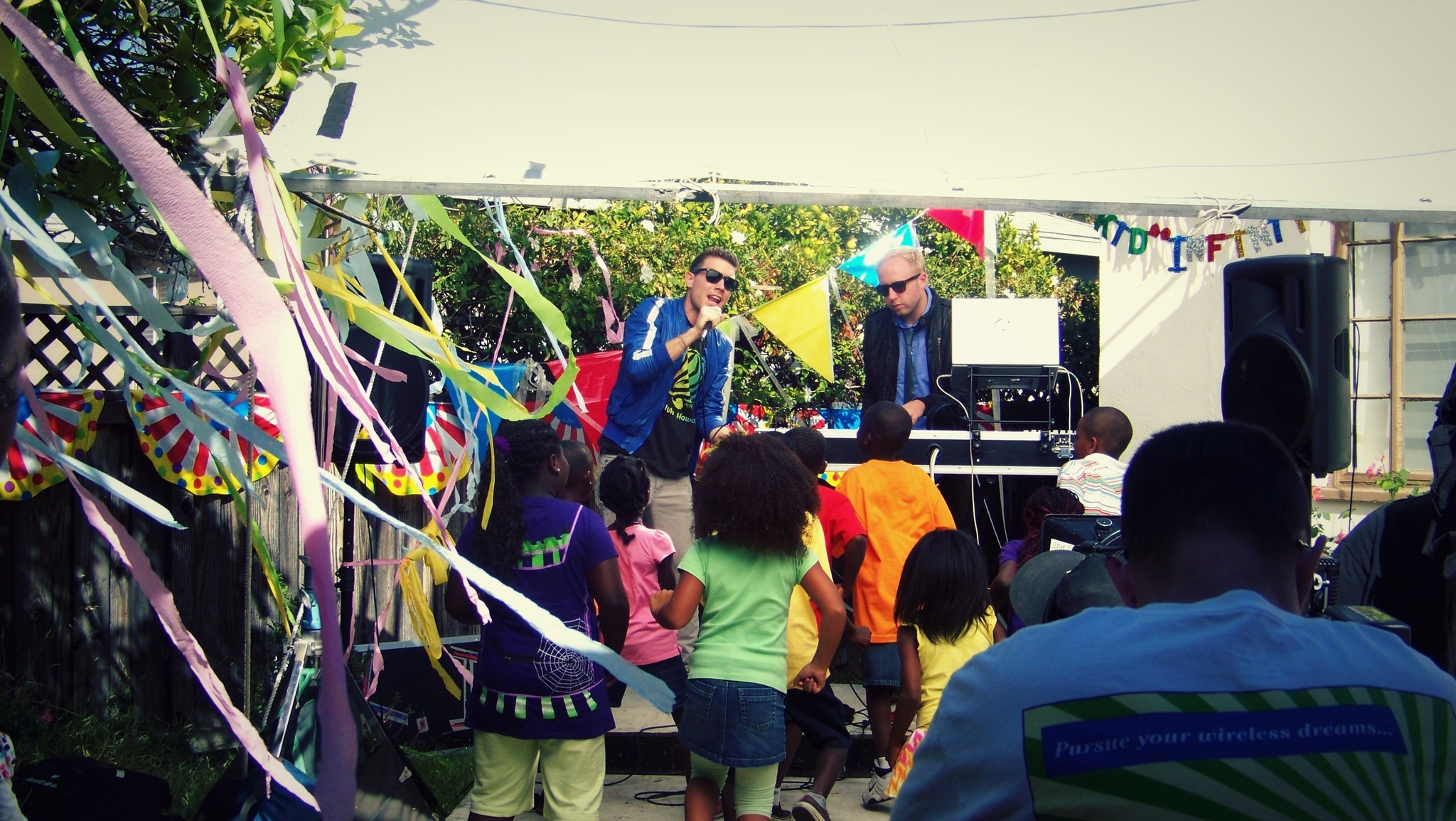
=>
[455,245,1456,821]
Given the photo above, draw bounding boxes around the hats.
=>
[1010,547,1128,631]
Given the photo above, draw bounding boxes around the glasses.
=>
[695,268,740,291]
[876,274,921,297]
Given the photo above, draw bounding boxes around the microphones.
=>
[698,304,722,354]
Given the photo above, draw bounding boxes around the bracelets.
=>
[677,334,688,351]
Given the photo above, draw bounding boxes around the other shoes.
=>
[791,793,832,821]
[714,799,725,818]
[770,801,793,819]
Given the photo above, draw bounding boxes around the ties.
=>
[899,326,920,405]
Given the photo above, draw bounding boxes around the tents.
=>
[211,1,1456,623]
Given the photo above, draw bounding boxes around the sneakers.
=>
[862,771,899,807]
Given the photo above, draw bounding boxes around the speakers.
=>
[1218,254,1352,477]
[315,251,433,465]
[343,633,483,749]
